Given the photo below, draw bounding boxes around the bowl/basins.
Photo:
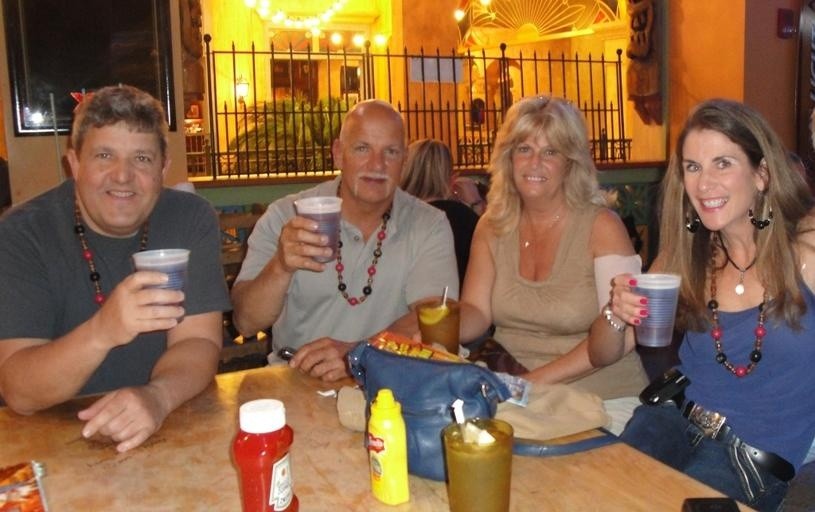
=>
[708,233,769,377]
[74,198,148,309]
[336,180,392,306]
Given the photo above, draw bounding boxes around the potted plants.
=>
[3,0,177,135]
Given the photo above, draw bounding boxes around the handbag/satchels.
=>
[471,199,483,212]
[604,305,626,333]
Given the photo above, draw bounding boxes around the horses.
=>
[278,347,297,360]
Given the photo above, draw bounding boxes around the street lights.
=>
[350,328,513,483]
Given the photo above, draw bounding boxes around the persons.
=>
[586,97,814,512]
[399,138,479,289]
[0,84,233,454]
[231,99,460,383]
[412,95,651,438]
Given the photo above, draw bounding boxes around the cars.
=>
[633,273,681,347]
[417,296,460,356]
[132,248,191,323]
[294,196,343,264]
[443,417,513,510]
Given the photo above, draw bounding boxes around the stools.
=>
[673,394,797,483]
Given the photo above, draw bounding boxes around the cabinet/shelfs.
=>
[230,398,300,512]
[365,386,410,506]
